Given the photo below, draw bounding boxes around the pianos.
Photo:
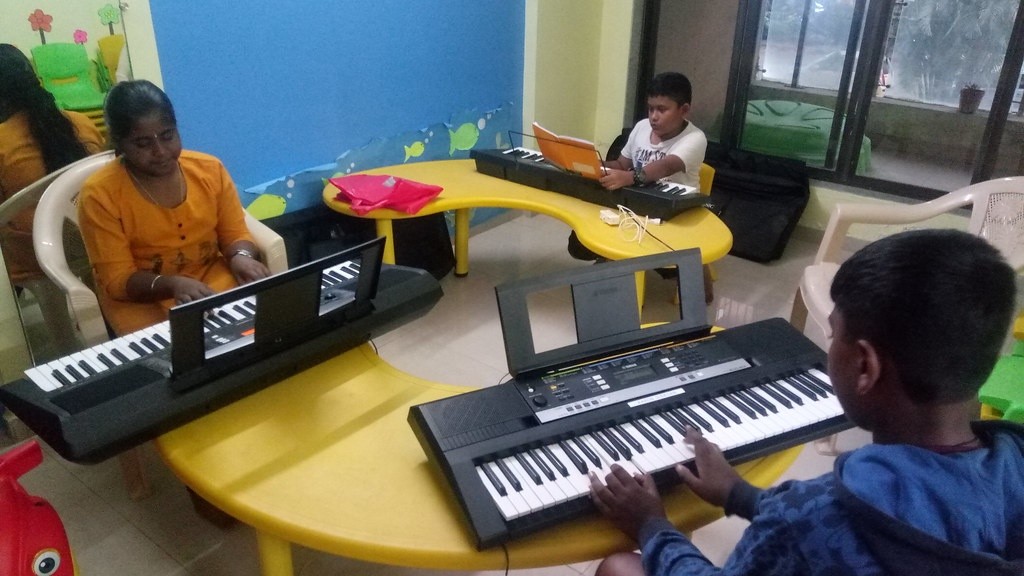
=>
[469,145,711,223]
[408,314,863,556]
[0,255,450,469]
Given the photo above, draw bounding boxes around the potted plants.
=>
[959,81,985,115]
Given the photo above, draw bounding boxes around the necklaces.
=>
[125,164,184,208]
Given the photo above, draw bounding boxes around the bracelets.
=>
[150,275,164,298]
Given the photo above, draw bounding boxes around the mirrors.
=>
[0,0,131,367]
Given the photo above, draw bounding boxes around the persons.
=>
[566,71,714,307]
[585,224,1024,575]
[0,42,105,299]
[81,79,271,336]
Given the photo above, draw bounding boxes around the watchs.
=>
[629,167,647,186]
[231,250,255,258]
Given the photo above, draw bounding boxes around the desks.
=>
[155,322,805,576]
[322,159,734,308]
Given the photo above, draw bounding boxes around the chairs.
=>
[0,150,115,358]
[30,34,126,141]
[32,155,289,502]
[699,163,718,282]
[788,177,1024,353]
[977,312,1024,425]
